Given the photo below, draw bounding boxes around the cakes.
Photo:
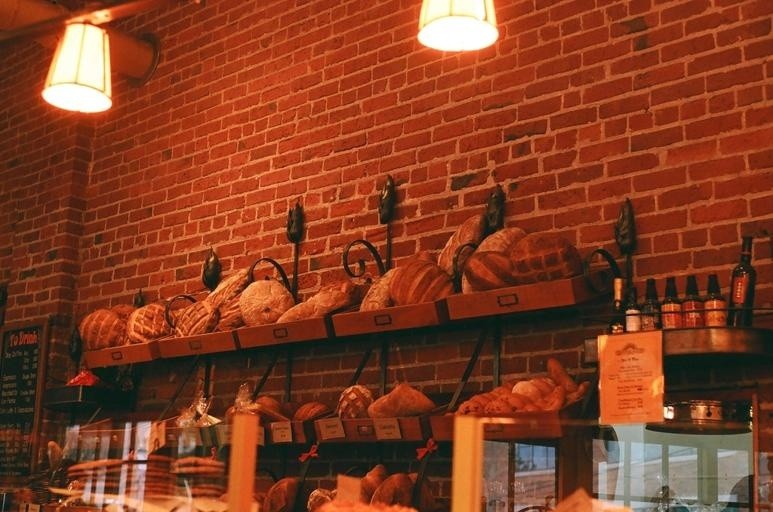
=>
[691,400,722,424]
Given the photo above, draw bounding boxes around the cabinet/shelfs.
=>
[606,234,757,335]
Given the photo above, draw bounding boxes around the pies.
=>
[64,451,225,500]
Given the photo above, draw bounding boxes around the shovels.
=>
[64,451,225,500]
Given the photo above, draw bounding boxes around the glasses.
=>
[37,19,113,114]
[415,1,500,55]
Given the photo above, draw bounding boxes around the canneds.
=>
[691,400,722,424]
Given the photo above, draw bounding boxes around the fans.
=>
[0,314,50,485]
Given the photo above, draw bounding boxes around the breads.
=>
[225,357,587,423]
[78,211,583,352]
[254,464,435,511]
[48,442,62,467]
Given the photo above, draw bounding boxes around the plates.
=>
[75,250,634,512]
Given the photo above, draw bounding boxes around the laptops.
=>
[254,464,435,511]
[48,442,62,467]
